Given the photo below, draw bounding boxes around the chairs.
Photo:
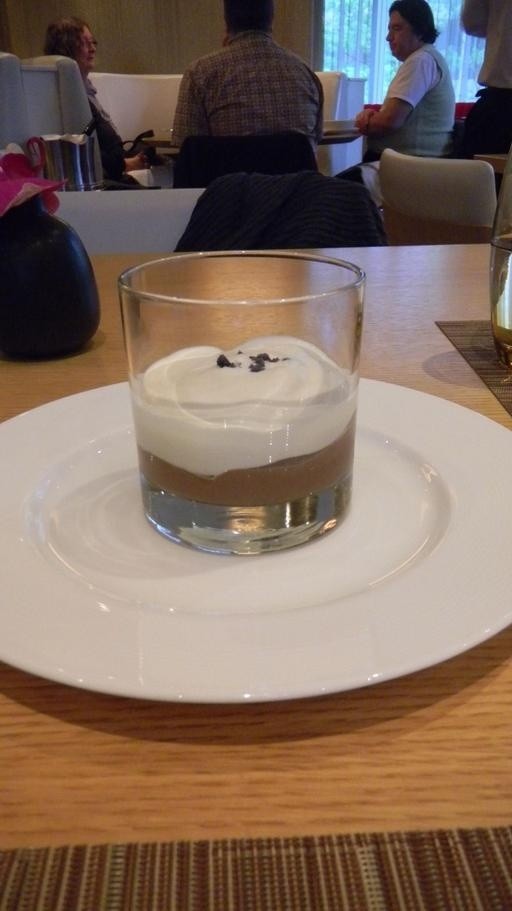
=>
[377,147,496,244]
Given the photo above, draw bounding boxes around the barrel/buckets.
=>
[38,133,97,193]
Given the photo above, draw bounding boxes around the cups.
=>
[118,251,368,561]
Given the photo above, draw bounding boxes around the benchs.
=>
[90,74,365,158]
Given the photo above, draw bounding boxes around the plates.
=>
[1,361,512,705]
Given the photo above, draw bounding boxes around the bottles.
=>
[488,144,512,366]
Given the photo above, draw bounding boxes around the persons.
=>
[332,0,456,210]
[171,0,326,166]
[42,14,165,190]
[459,0,510,158]
